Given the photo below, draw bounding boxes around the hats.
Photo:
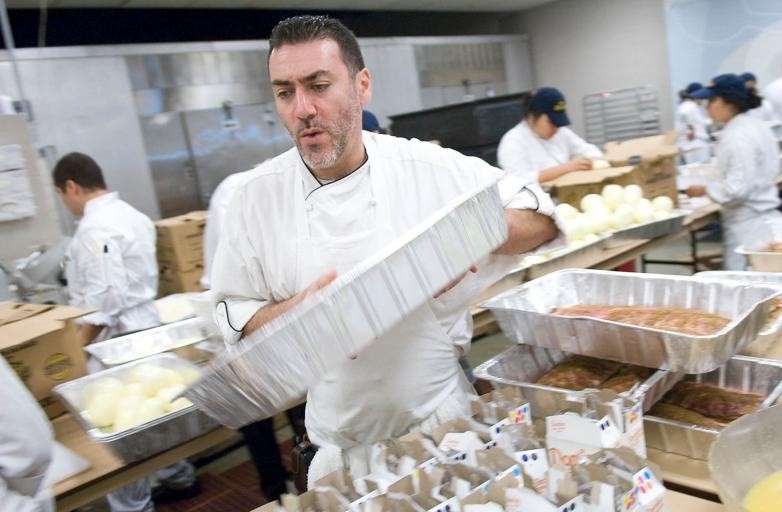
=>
[529,88,569,126]
[686,73,755,98]
[363,111,387,131]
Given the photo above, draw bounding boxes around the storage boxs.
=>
[0,300,100,424]
[540,128,703,213]
[151,211,207,302]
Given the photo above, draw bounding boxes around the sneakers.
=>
[152,476,201,503]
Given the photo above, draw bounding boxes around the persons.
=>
[0,352,58,511]
[191,17,569,496]
[51,152,214,511]
[686,73,782,271]
[236,402,304,503]
[496,88,605,210]
[674,83,719,241]
[742,72,782,168]
